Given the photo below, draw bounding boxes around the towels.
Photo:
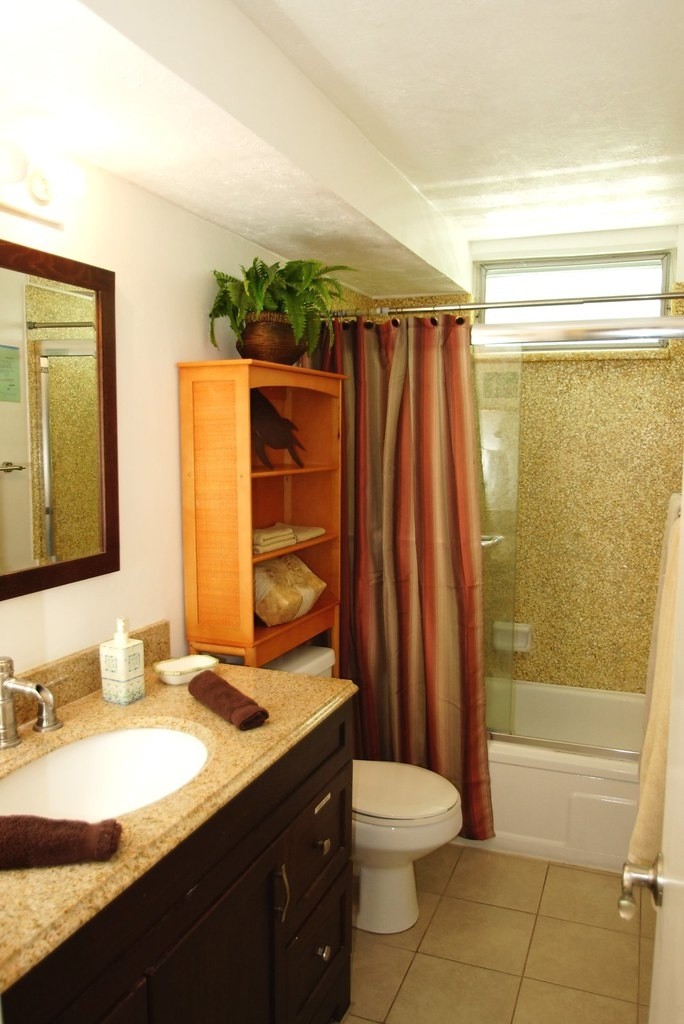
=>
[0,814,123,872]
[252,521,326,555]
[187,670,269,731]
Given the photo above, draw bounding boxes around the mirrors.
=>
[0,239,120,602]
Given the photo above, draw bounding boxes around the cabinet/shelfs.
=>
[0,696,355,1024]
[177,358,348,677]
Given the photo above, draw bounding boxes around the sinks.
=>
[0,727,209,833]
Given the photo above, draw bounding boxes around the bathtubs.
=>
[420,676,649,877]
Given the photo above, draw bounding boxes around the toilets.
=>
[353,760,464,934]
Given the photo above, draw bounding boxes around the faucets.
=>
[0,656,63,750]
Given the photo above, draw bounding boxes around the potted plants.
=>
[209,258,359,366]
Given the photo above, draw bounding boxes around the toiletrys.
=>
[99,617,146,705]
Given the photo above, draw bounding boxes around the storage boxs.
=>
[259,644,336,679]
[252,555,327,625]
